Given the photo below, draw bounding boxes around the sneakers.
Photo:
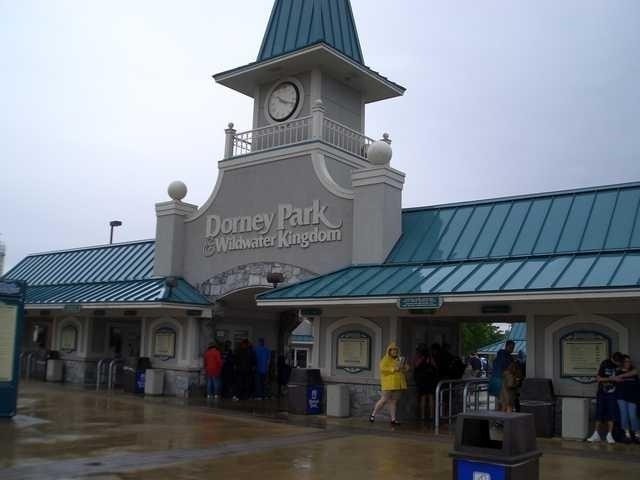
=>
[586,429,640,444]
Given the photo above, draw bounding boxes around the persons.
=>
[615,355,640,440]
[203,337,289,401]
[586,352,639,444]
[369,340,526,424]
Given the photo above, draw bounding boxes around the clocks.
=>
[267,80,300,122]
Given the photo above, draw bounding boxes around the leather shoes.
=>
[369,413,402,425]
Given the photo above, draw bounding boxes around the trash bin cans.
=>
[46,360,63,381]
[520,379,556,437]
[136,358,150,393]
[145,370,164,395]
[562,398,589,442]
[287,368,324,415]
[448,413,543,479]
[193,383,206,399]
[326,385,350,416]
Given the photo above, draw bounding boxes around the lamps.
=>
[165,275,179,298]
[109,219,122,244]
[266,271,284,289]
